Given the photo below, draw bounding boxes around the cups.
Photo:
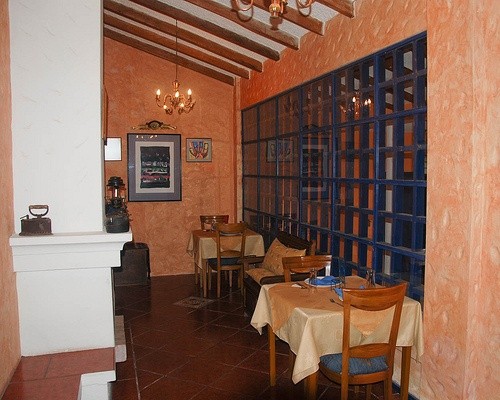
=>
[309,269,317,295]
[381,273,393,288]
[366,267,376,289]
[331,279,341,292]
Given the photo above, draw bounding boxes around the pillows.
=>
[244,268,276,287]
[262,237,307,275]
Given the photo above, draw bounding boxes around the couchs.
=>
[236,231,317,316]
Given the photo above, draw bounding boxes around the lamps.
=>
[155,18,197,115]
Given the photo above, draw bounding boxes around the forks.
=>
[329,298,344,307]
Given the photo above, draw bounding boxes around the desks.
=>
[193,228,263,298]
[263,274,420,400]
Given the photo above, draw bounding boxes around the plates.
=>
[222,233,239,237]
[339,296,344,302]
[304,277,342,287]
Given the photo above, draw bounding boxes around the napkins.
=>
[309,276,342,286]
[334,285,365,298]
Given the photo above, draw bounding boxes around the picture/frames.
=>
[185,138,213,163]
[266,138,295,163]
[127,133,182,202]
[297,137,341,201]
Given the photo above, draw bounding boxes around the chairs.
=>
[208,222,248,298]
[304,281,409,400]
[199,215,230,288]
[281,254,332,380]
